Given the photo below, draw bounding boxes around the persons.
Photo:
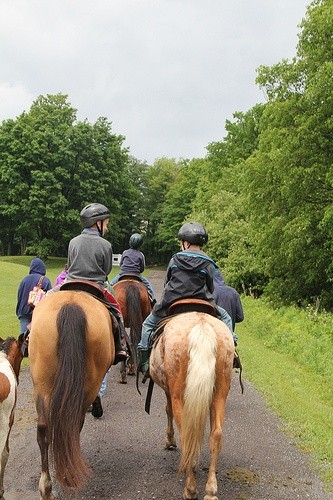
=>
[16,258,52,334]
[136,222,238,371]
[24,203,130,359]
[87,367,111,418]
[55,263,68,286]
[110,233,157,309]
[212,269,244,332]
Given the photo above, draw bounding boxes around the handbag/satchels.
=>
[27,276,46,309]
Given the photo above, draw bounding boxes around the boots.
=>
[138,350,149,372]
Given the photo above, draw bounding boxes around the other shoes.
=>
[117,342,131,358]
[87,396,103,417]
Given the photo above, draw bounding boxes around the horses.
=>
[0,333,25,499]
[104,277,154,386]
[146,296,234,500]
[28,281,114,500]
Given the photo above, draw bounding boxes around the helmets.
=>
[178,222,208,244]
[80,203,111,227]
[130,233,143,248]
